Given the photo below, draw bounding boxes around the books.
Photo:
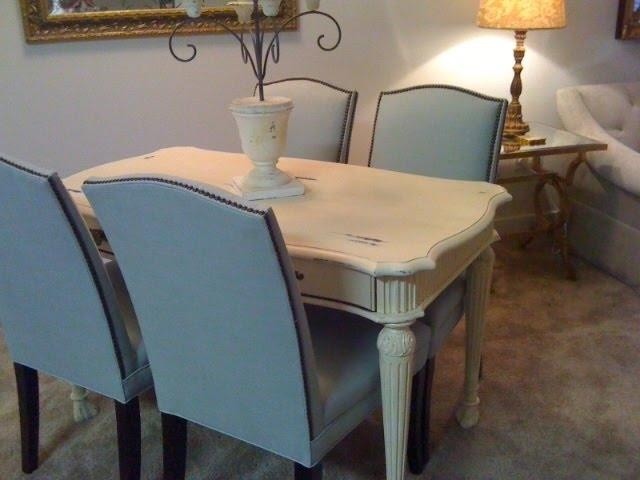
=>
[232,174,304,200]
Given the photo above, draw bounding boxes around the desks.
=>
[499,119,607,281]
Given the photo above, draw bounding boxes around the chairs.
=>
[81,172,424,479]
[253,77,358,164]
[556,81,640,288]
[368,84,508,466]
[1,153,153,479]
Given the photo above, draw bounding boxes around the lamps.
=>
[475,0,566,145]
[169,0,342,200]
